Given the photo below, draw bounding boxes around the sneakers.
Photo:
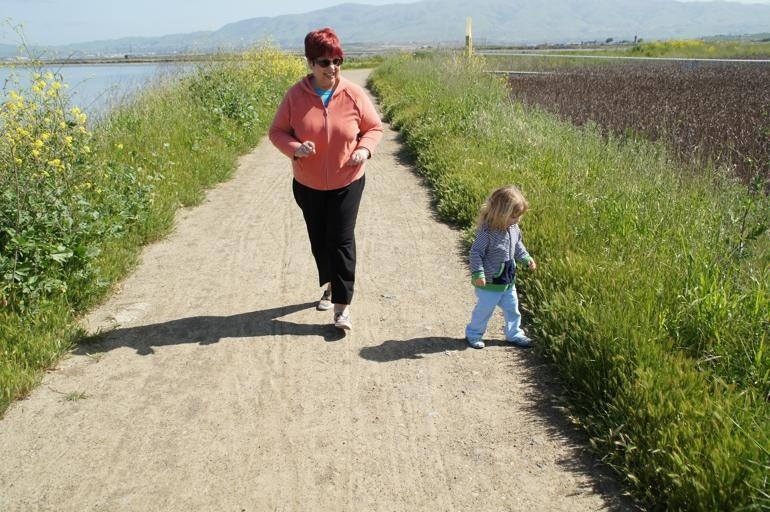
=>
[467,336,484,348]
[513,336,534,347]
[318,290,334,310]
[334,312,352,330]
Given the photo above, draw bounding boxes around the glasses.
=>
[317,58,341,68]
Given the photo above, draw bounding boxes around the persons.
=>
[269,27,383,330]
[465,185,536,349]
